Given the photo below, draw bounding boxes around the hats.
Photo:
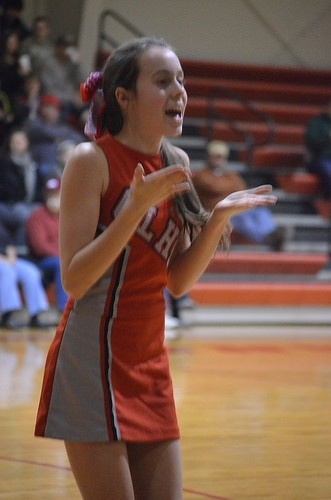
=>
[40,96,60,107]
[207,140,229,156]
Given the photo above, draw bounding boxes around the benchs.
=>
[0,278,77,328]
[96,52,330,326]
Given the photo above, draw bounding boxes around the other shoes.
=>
[31,313,61,327]
[3,312,29,327]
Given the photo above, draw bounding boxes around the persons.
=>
[0,1,94,327]
[192,140,286,252]
[34,39,278,500]
